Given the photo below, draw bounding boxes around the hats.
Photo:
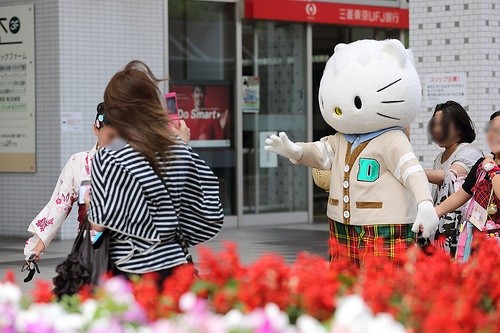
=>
[312,168,330,192]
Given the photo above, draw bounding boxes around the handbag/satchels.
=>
[52,218,116,303]
[176,233,193,262]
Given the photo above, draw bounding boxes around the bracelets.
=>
[484,161,500,180]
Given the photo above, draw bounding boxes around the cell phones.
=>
[164,92,179,130]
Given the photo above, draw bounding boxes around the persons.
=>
[419,112,500,264]
[24,103,119,262]
[84,61,224,283]
[186,85,230,140]
[423,101,484,260]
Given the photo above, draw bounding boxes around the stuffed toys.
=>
[264,38,439,271]
